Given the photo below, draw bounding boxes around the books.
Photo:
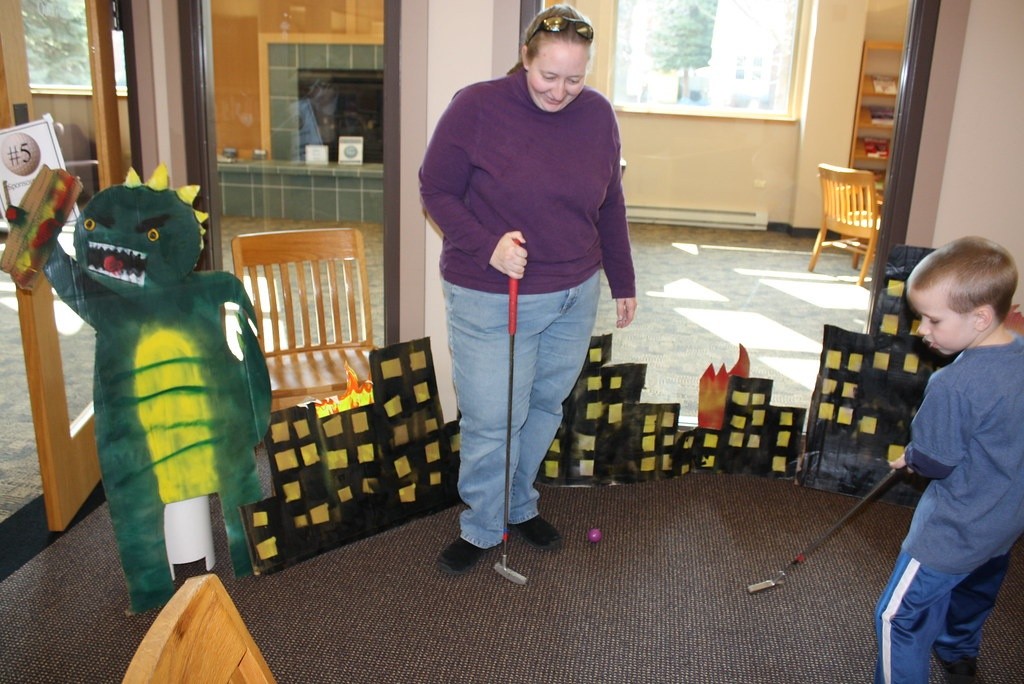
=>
[873,74,896,94]
[865,138,888,159]
[870,106,894,126]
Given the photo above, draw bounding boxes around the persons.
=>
[872,236,1024,684]
[419,4,637,571]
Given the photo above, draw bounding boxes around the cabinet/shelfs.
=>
[841,37,905,245]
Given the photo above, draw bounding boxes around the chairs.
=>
[56,123,102,202]
[231,227,380,411]
[120,574,278,684]
[806,162,880,287]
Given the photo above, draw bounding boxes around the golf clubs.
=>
[493,236,528,586]
[746,467,909,595]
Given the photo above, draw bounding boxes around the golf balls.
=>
[588,528,601,542]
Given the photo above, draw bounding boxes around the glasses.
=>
[525,15,594,46]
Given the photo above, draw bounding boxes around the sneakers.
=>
[437,537,502,576]
[507,514,562,549]
